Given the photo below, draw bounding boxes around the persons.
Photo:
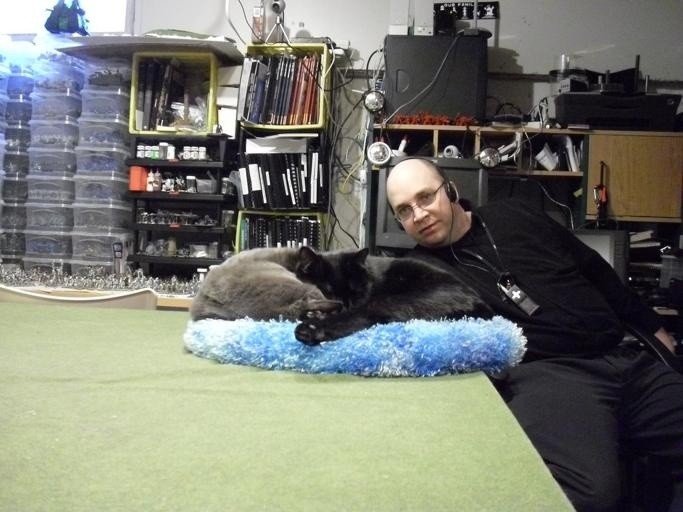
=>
[384,156,682,510]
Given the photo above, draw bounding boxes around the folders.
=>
[238,152,328,208]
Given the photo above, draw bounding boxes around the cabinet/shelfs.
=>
[587,130,683,223]
[121,134,226,281]
[369,123,584,260]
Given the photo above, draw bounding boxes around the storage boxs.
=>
[234,39,332,259]
[0,72,132,281]
[130,47,218,134]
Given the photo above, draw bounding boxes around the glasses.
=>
[393,181,446,224]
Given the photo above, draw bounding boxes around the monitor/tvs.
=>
[374,157,489,249]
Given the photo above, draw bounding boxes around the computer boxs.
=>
[382,33,488,125]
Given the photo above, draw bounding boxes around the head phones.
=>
[387,158,457,232]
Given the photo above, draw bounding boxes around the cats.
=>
[292,245,495,347]
[189,245,359,322]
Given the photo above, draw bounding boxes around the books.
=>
[136,58,182,133]
[560,136,583,172]
[243,55,321,250]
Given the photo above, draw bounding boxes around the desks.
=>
[1,308,579,510]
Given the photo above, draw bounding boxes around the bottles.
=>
[185,175,196,189]
[145,168,162,192]
[158,141,168,159]
